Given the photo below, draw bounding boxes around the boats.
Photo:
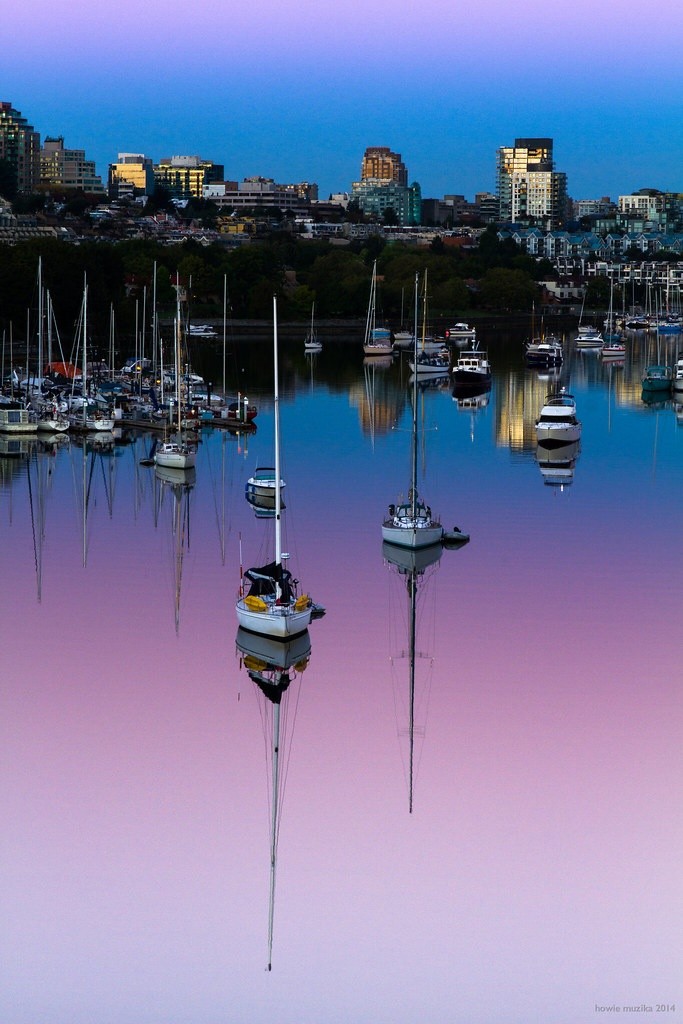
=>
[245,491,287,518]
[534,440,581,486]
[536,385,582,445]
[248,465,286,497]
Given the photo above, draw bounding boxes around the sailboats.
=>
[0,252,259,469]
[362,257,683,394]
[1,418,258,639]
[236,624,313,974]
[380,540,442,814]
[382,272,471,552]
[362,354,683,477]
[237,294,327,641]
